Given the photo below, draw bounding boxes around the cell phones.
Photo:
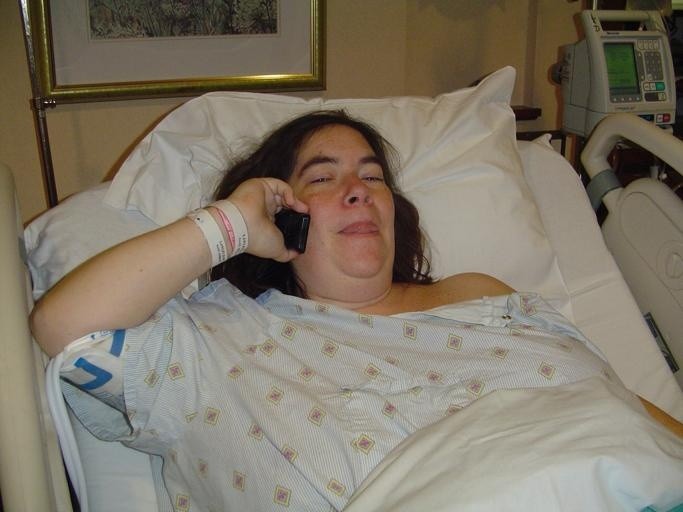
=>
[274,207,311,253]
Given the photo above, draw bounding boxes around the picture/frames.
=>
[31,0,326,101]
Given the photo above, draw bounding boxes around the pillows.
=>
[103,66,572,315]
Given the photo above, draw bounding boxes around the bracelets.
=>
[187,208,228,269]
[207,197,249,262]
